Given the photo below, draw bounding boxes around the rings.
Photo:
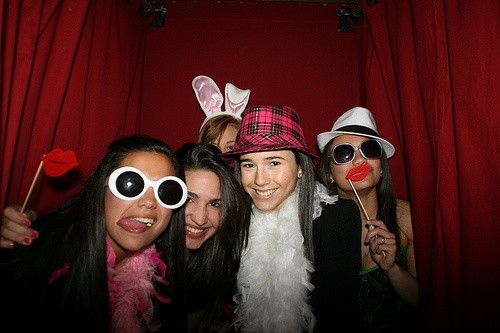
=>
[382,237,385,244]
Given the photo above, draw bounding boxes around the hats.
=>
[317,107,395,159]
[219,106,320,169]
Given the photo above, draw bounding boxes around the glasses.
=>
[104,166,188,210]
[329,139,382,164]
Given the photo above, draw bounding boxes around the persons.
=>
[309,106,425,333]
[0,140,246,333]
[0,133,192,333]
[191,75,252,161]
[215,103,365,332]
